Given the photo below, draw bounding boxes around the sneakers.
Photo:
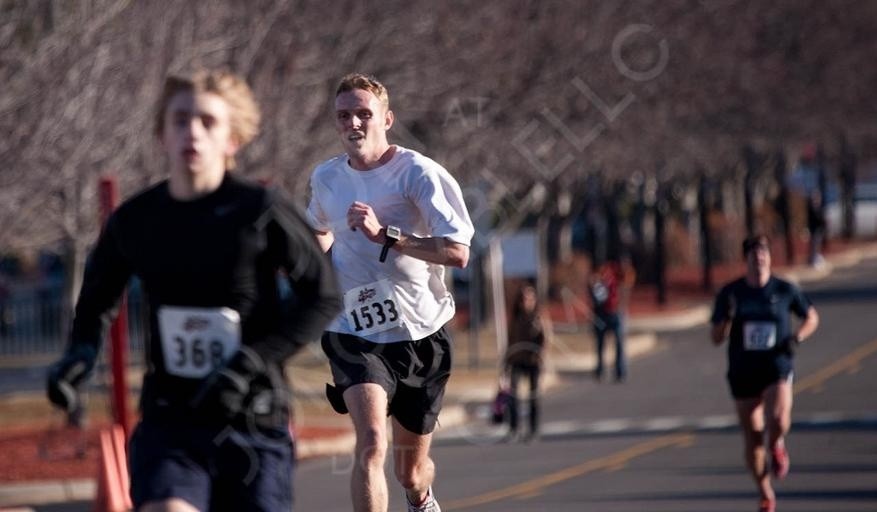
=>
[406,482,442,512]
[768,428,791,480]
[759,496,777,511]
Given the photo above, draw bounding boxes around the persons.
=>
[581,248,637,383]
[42,65,346,512]
[708,234,822,512]
[501,283,553,444]
[798,191,828,273]
[288,71,478,512]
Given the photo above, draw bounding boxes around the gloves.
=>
[45,330,103,413]
[770,335,801,361]
[718,290,736,321]
[194,344,265,432]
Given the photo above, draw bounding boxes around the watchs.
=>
[377,224,401,263]
